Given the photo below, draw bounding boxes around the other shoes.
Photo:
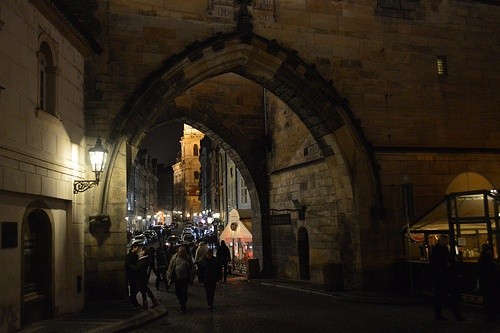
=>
[207,306,213,312]
[459,317,466,320]
[436,316,446,318]
[180,309,186,313]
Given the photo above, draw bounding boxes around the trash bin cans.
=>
[246,257,261,282]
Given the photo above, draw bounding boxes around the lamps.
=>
[73,138,109,195]
